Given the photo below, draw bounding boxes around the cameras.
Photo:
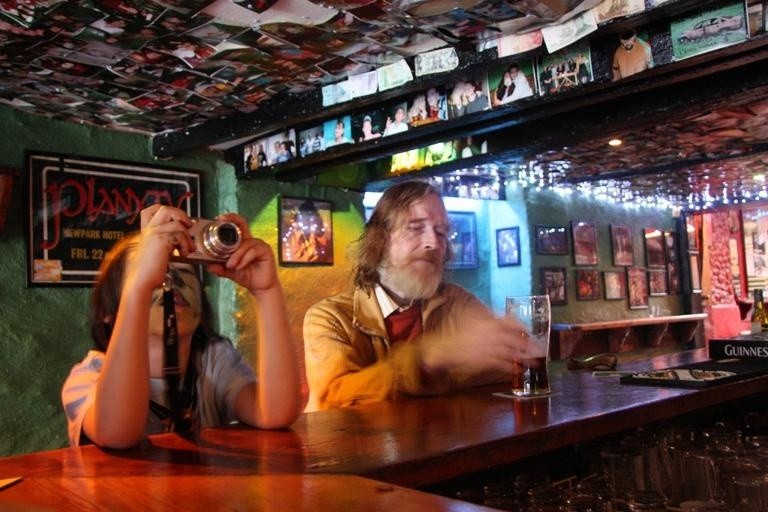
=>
[168,217,245,263]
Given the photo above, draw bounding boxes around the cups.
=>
[452,410,768,511]
[504,293,553,400]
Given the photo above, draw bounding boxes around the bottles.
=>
[747,287,768,328]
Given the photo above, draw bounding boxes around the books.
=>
[620,360,768,388]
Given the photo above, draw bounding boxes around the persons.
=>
[62,203,301,450]
[303,183,542,413]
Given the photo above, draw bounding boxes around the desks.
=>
[551,312,708,360]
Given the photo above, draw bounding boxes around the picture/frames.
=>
[575,269,601,302]
[443,210,480,271]
[496,225,521,267]
[23,147,209,289]
[600,210,702,310]
[570,219,600,266]
[535,223,569,256]
[276,192,335,266]
[540,267,569,305]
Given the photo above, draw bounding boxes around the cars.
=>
[677,13,744,44]
